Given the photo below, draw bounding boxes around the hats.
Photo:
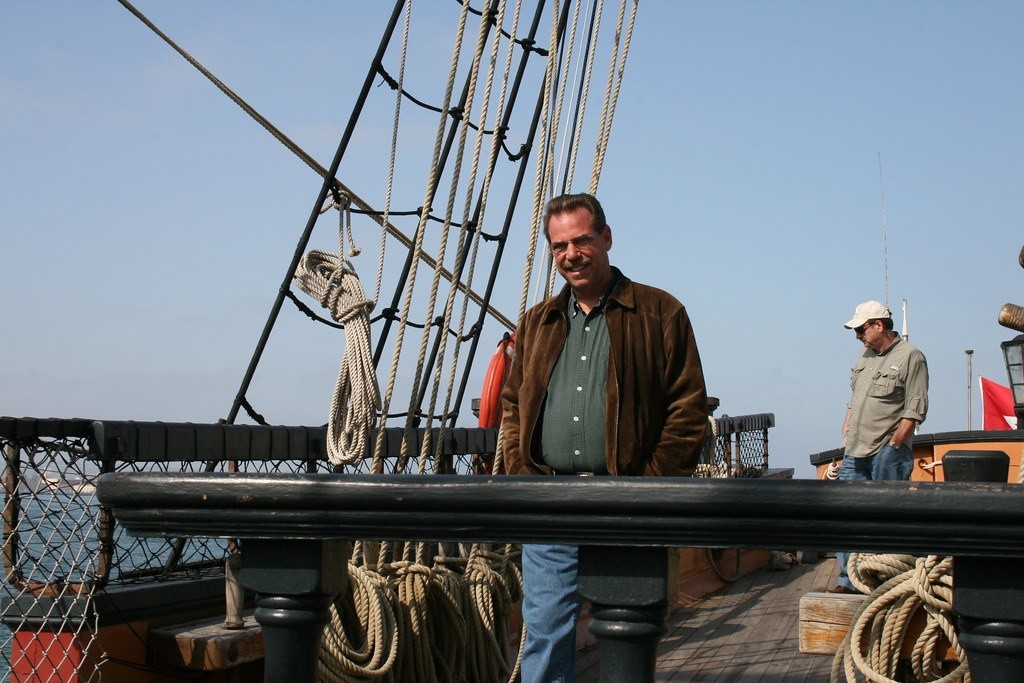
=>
[844,300,891,330]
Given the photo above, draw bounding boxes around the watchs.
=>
[890,442,900,449]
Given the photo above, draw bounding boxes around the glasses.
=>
[547,231,602,256]
[854,323,875,335]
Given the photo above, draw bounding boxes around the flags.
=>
[979,376,1018,431]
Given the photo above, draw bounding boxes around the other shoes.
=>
[825,585,863,594]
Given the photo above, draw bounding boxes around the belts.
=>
[539,465,610,478]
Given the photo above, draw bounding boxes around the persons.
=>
[825,301,930,594]
[501,193,710,683]
